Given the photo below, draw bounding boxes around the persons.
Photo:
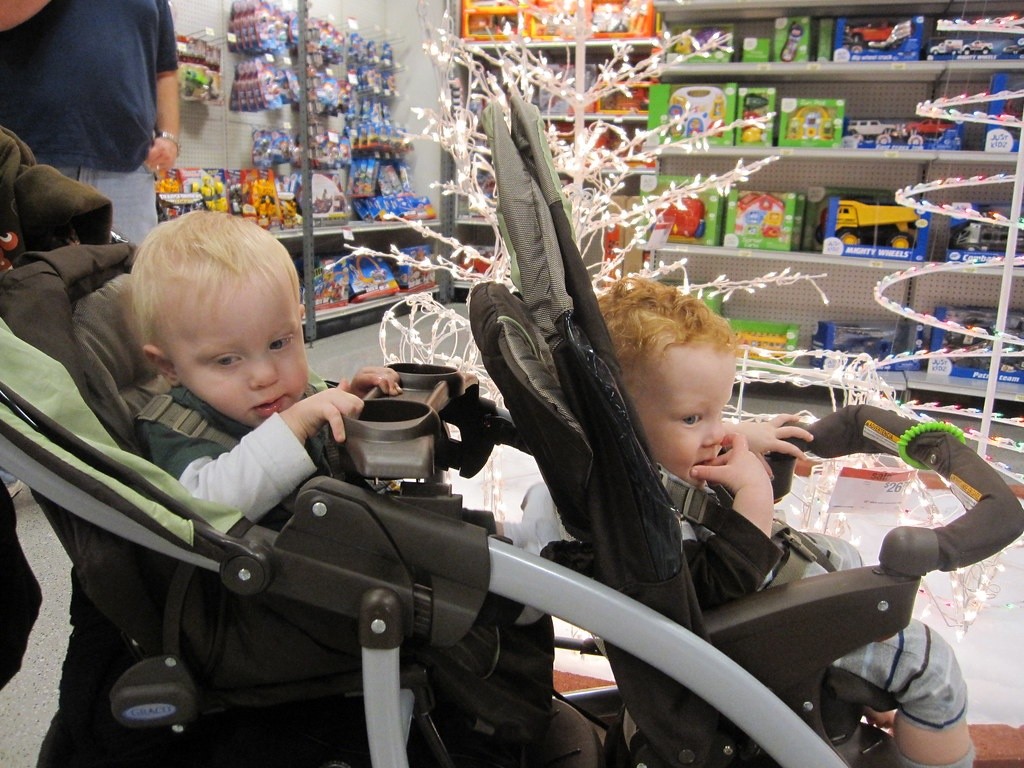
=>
[157,170,180,220]
[135,212,562,624]
[0,0,181,246]
[1,124,115,686]
[229,172,296,230]
[191,177,228,213]
[593,274,977,768]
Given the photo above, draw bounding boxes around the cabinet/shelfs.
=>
[267,218,447,345]
[450,0,1024,401]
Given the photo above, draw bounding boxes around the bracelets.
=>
[157,132,180,156]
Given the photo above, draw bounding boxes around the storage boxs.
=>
[463,1,1023,387]
[293,246,437,313]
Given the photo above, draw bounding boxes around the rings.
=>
[156,166,159,169]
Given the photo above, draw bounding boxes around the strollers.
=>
[1,80,1023,768]
